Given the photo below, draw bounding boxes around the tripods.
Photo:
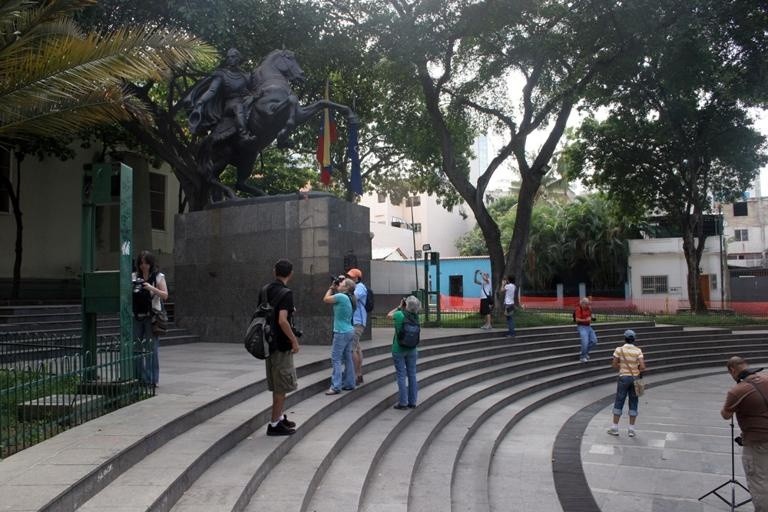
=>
[698,418,754,512]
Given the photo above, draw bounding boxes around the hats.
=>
[347,268,362,278]
[624,329,636,338]
[403,295,420,310]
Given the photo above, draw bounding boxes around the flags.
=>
[346,93,362,196]
[316,78,337,185]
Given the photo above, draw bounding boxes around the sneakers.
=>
[394,403,408,409]
[408,404,416,408]
[586,353,591,359]
[267,421,296,435]
[580,358,588,363]
[325,388,338,395]
[280,414,295,427]
[356,375,363,385]
[607,428,619,434]
[628,429,635,436]
[481,323,492,329]
[343,385,353,390]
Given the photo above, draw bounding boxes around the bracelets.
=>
[397,306,401,311]
[329,286,335,290]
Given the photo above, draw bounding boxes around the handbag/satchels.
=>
[151,308,168,336]
[503,306,515,316]
[488,295,494,304]
[634,379,645,396]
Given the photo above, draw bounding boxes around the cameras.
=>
[326,270,340,287]
[400,296,409,306]
[291,325,302,340]
[131,277,146,294]
[590,313,599,323]
[337,270,348,276]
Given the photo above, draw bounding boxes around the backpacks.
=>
[244,283,292,360]
[359,288,374,312]
[573,306,582,322]
[395,310,420,348]
[133,273,156,321]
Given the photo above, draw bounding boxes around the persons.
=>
[575,297,598,362]
[500,274,518,337]
[474,270,492,330]
[183,47,257,144]
[606,329,645,437]
[720,356,768,512]
[386,295,421,410]
[131,251,169,387]
[323,274,355,395]
[347,268,368,387]
[257,258,299,435]
